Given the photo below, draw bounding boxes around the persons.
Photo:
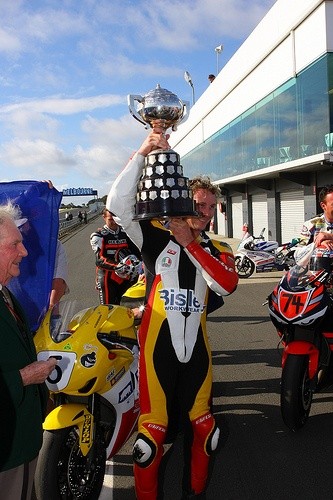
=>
[294,185,333,267]
[208,74,215,83]
[0,206,56,500]
[65,210,88,225]
[89,209,142,305]
[3,180,71,336]
[105,132,240,500]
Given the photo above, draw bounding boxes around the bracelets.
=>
[18,190,29,203]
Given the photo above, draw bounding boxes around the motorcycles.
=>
[32,279,146,499]
[238,222,278,252]
[263,253,333,434]
[233,232,310,278]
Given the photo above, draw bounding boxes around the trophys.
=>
[127,83,202,222]
